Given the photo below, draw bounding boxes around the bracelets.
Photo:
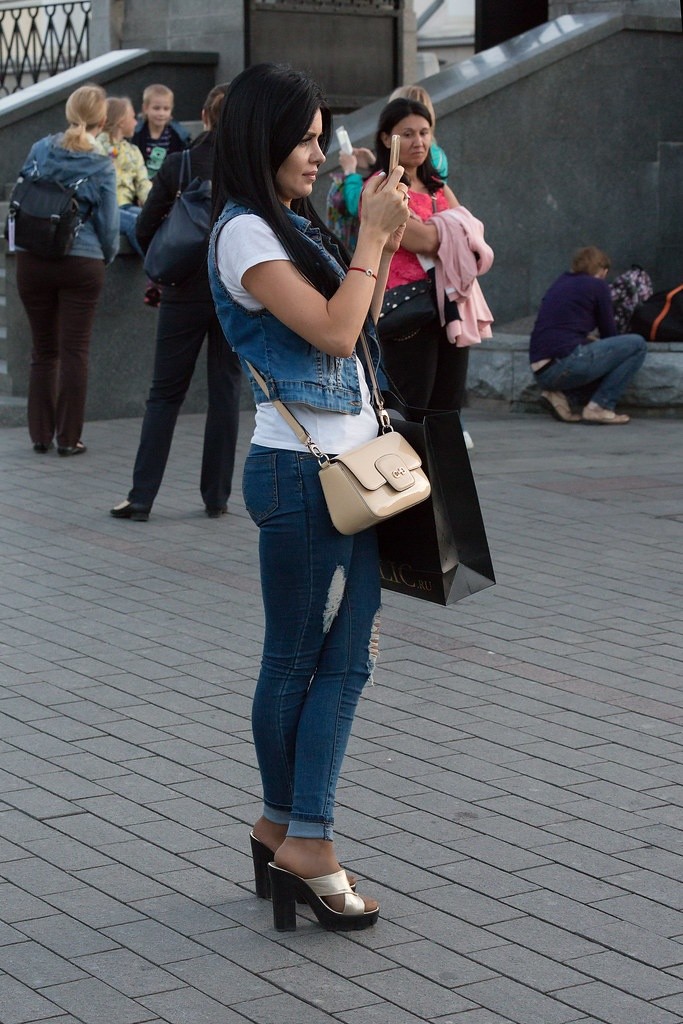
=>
[348,267,378,281]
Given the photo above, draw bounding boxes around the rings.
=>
[399,189,410,199]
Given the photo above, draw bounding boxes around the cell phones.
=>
[336,126,353,157]
[389,134,401,176]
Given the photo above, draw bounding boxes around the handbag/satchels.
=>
[327,170,362,256]
[367,312,496,607]
[635,283,683,344]
[145,147,217,289]
[377,277,436,341]
[318,431,432,535]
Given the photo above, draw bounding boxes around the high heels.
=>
[268,861,379,932]
[249,831,356,899]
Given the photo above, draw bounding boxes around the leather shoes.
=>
[109,502,152,522]
[583,406,630,424]
[540,389,582,423]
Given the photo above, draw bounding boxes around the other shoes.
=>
[32,441,55,453]
[204,503,229,518]
[144,280,162,308]
[58,441,86,457]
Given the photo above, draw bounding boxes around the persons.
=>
[106,85,191,262]
[113,83,241,520]
[526,246,647,424]
[326,91,491,452]
[208,64,413,932]
[7,85,121,455]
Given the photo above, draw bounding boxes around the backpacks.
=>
[3,153,93,258]
[596,264,653,335]
